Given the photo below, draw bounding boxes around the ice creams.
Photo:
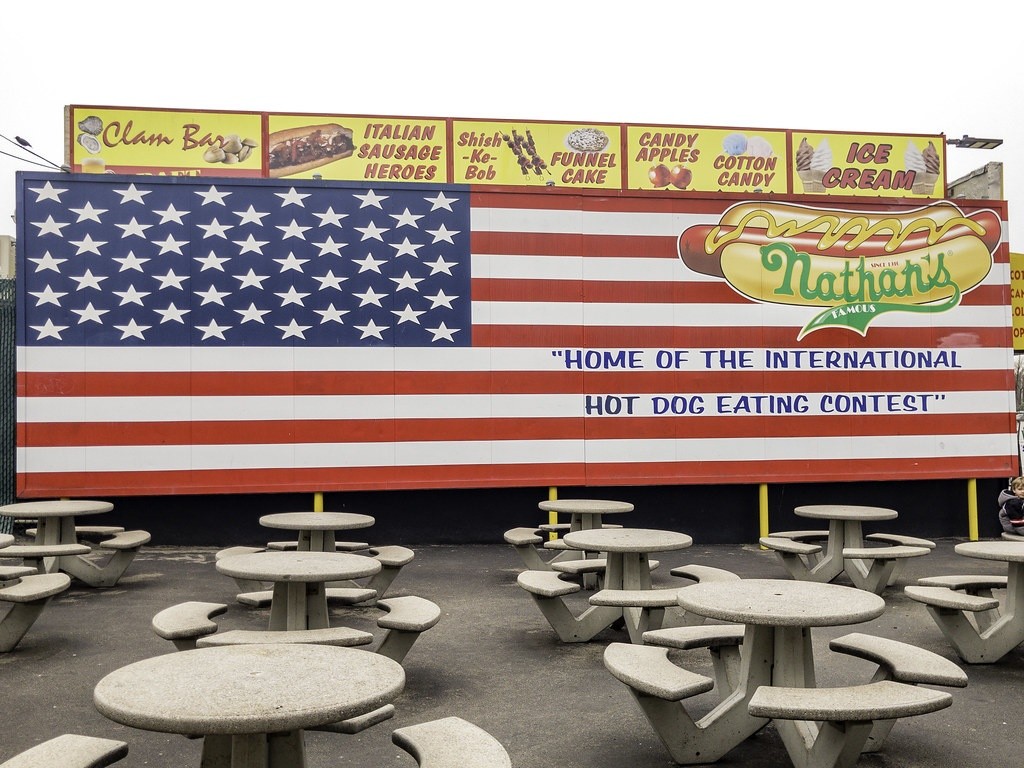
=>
[903,140,940,196]
[795,136,832,192]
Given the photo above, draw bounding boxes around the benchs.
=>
[0,524,1024,768]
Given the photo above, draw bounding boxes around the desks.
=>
[954,541,1024,663]
[794,505,898,596]
[563,528,693,646]
[260,512,377,551]
[0,501,114,589]
[93,642,406,768]
[537,499,634,592]
[677,579,886,768]
[215,550,382,632]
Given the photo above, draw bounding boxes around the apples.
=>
[648,163,693,189]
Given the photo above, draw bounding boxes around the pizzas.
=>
[568,129,609,153]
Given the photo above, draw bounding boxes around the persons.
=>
[998,477,1024,536]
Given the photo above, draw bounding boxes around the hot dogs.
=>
[268,123,355,175]
